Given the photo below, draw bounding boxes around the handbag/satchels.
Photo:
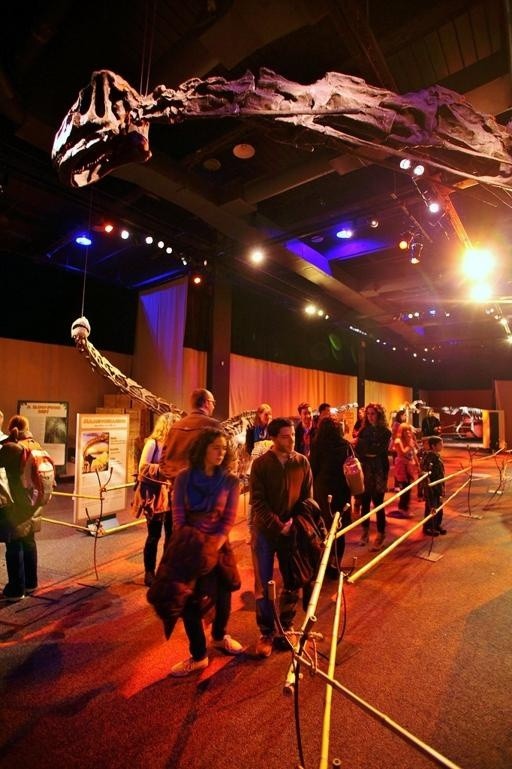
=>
[342,453,365,494]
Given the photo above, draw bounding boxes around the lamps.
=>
[397,221,426,265]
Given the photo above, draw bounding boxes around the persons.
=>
[172,426,244,677]
[160,389,228,488]
[247,416,314,658]
[316,403,330,427]
[355,403,391,551]
[392,423,419,517]
[0,415,44,604]
[244,405,275,455]
[292,401,319,460]
[388,410,406,452]
[352,408,367,438]
[139,411,184,588]
[312,416,355,578]
[417,436,448,537]
[420,407,442,437]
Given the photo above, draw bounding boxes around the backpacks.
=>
[17,440,56,506]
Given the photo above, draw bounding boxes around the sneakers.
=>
[423,523,449,537]
[254,628,276,659]
[372,530,388,551]
[358,525,369,547]
[170,652,210,678]
[211,633,244,655]
[279,620,298,652]
[25,585,38,594]
[0,586,25,601]
[400,506,417,518]
[144,571,157,586]
